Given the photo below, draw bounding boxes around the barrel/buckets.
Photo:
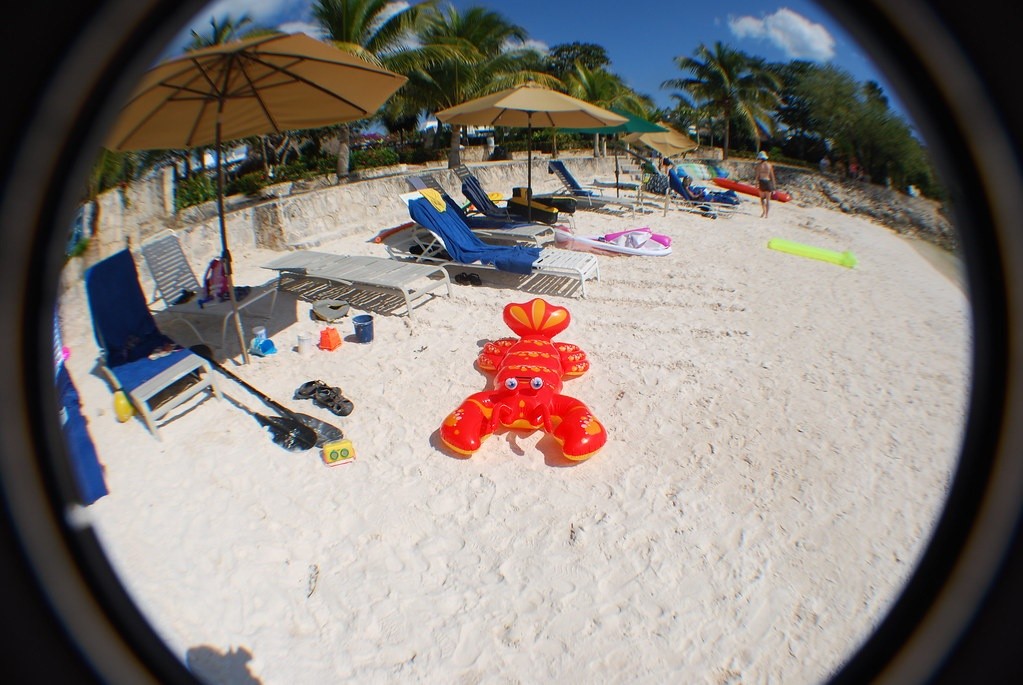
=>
[298,331,313,355]
[352,314,374,342]
[250,338,274,357]
[487,136,494,146]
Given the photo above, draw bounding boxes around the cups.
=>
[252,326,267,338]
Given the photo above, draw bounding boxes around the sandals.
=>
[315,387,354,416]
[296,380,342,399]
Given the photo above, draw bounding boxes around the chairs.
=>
[548,161,645,219]
[406,174,555,248]
[140,228,278,362]
[386,191,601,298]
[82,248,223,444]
[452,164,577,234]
[669,169,740,219]
[259,249,454,320]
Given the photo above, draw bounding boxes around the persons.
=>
[755,151,777,218]
[683,176,739,202]
[820,153,864,181]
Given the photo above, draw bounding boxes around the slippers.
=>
[467,273,483,285]
[455,272,471,285]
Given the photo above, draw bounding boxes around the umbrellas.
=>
[98,32,409,262]
[556,106,671,198]
[434,79,629,221]
[621,120,700,158]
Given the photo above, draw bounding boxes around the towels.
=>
[408,197,544,275]
[669,168,739,204]
[419,188,447,213]
[441,194,535,229]
[84,247,194,404]
[548,160,599,197]
[462,176,535,219]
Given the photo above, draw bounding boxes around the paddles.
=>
[189,368,319,454]
[189,342,345,451]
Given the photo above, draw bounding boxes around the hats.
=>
[756,150,769,160]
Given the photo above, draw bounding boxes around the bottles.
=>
[203,256,228,298]
[114,390,131,423]
